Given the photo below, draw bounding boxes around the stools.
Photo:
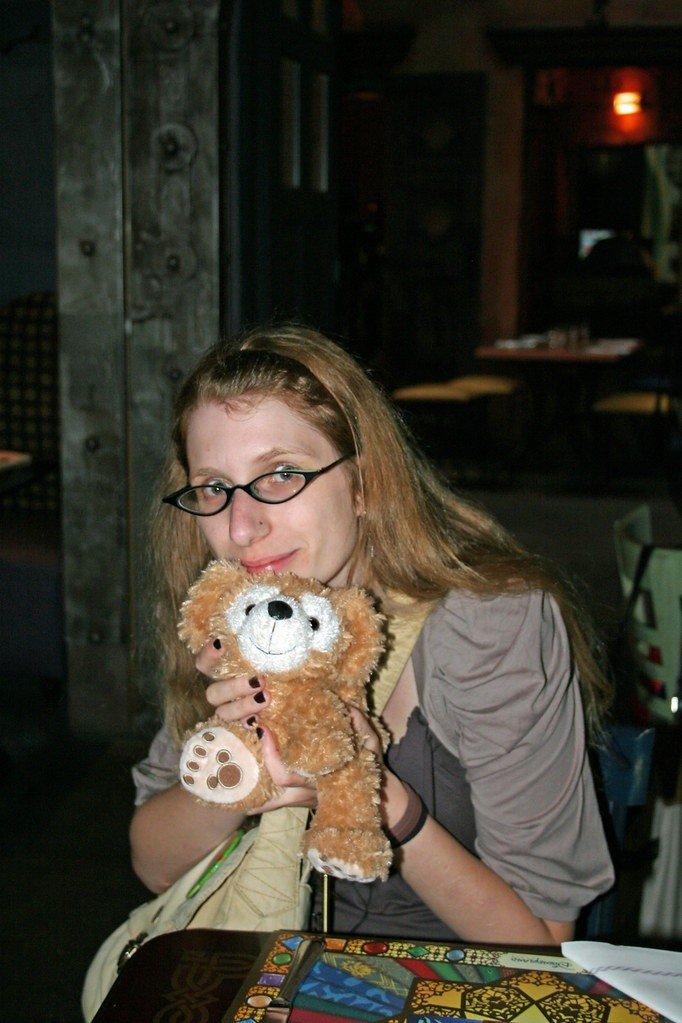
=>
[395,376,670,493]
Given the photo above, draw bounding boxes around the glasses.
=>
[162,453,356,516]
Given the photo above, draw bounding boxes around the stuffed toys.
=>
[174,554,399,884]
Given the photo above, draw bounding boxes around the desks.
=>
[474,340,638,470]
[81,927,682,1023]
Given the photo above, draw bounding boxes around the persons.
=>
[128,321,619,946]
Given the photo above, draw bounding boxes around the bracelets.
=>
[387,779,424,845]
